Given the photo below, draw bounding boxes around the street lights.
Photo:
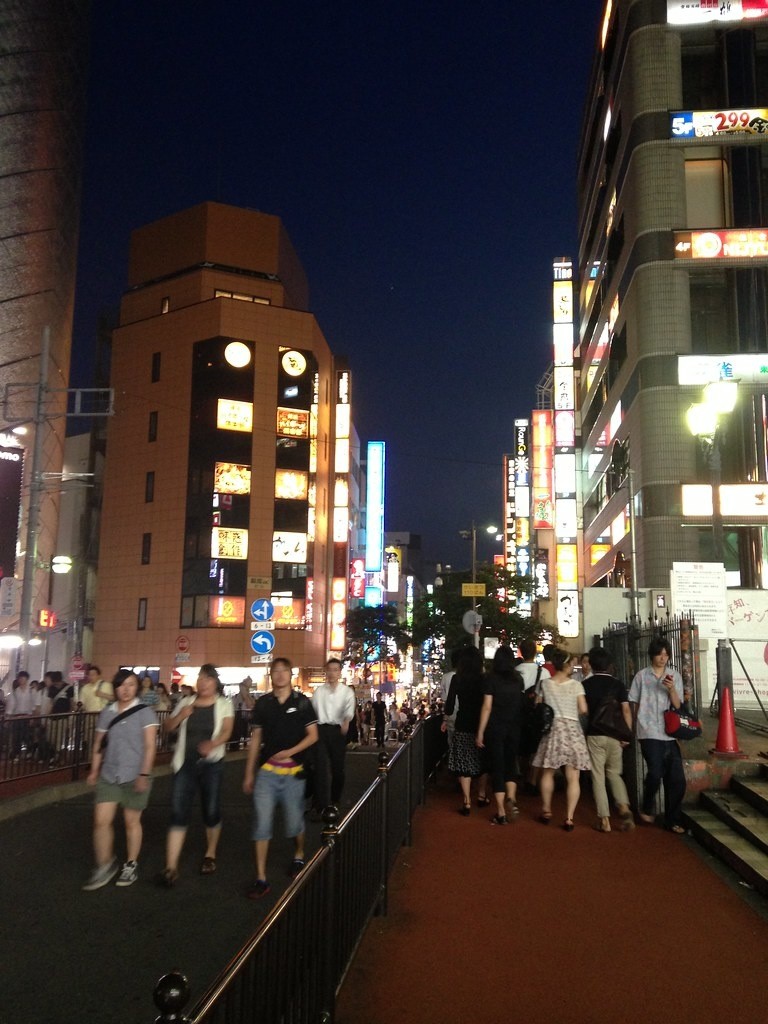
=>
[39,554,76,685]
[459,518,498,653]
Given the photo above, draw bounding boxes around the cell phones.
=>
[666,674,673,680]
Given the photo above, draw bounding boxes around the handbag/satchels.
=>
[532,681,555,735]
[664,702,702,741]
[524,664,542,699]
[591,678,633,744]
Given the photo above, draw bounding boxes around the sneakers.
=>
[251,877,271,898]
[83,860,117,891]
[290,859,304,878]
[116,860,138,887]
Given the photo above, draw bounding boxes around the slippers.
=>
[620,819,635,831]
[591,822,611,834]
[642,813,653,823]
[667,824,685,834]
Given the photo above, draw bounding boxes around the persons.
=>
[242,657,319,900]
[156,663,234,884]
[81,669,161,891]
[347,684,444,750]
[440,639,636,832]
[311,658,356,824]
[0,666,255,768]
[626,638,687,834]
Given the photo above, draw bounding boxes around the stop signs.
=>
[170,671,181,684]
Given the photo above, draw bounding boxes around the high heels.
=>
[566,818,575,832]
[463,802,471,815]
[478,794,490,807]
[540,812,552,824]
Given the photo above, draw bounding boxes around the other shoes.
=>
[202,857,217,873]
[157,868,179,882]
[491,813,508,826]
[509,802,519,816]
[9,751,58,769]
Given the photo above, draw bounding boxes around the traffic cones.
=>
[708,687,751,758]
